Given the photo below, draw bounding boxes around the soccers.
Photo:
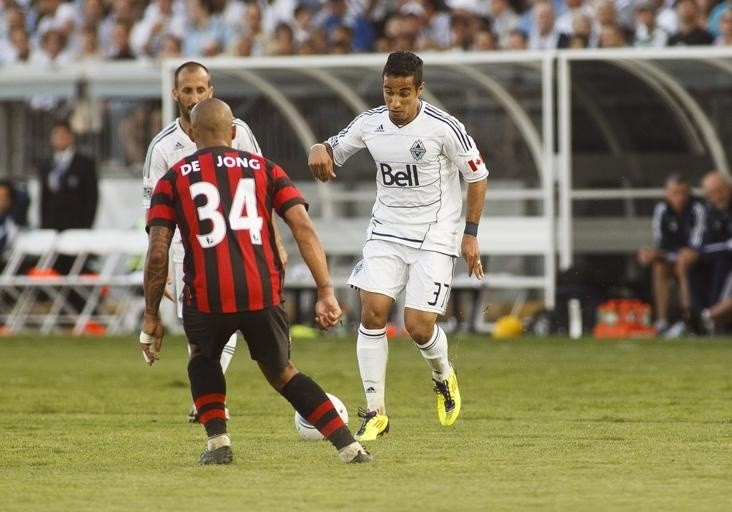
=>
[493,317,524,342]
[294,392,349,443]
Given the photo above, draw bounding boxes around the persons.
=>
[139,98,372,466]
[306,48,490,440]
[141,60,289,426]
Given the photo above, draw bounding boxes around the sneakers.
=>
[197,445,234,465]
[432,368,463,426]
[187,402,229,424]
[353,406,390,442]
[337,442,373,464]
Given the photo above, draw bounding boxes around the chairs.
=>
[0,229,147,337]
